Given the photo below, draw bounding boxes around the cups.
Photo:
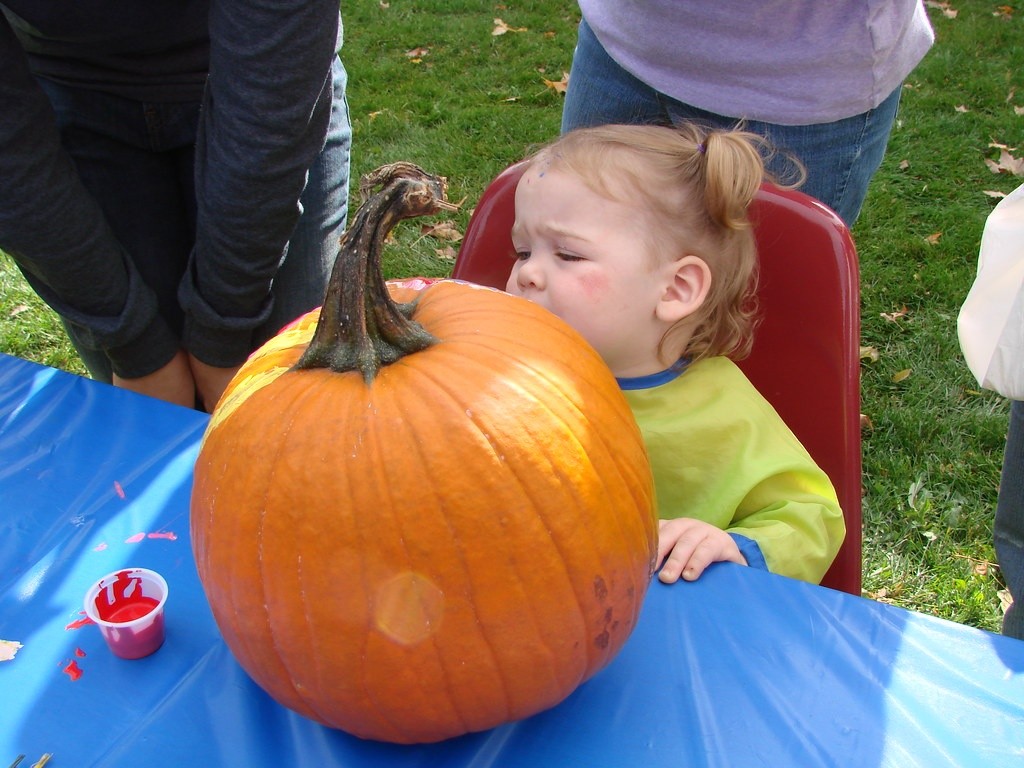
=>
[85,568,169,659]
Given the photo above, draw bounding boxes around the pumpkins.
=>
[190,160,658,744]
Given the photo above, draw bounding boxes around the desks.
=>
[0,354,1024,767]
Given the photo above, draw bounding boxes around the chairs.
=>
[453,155,863,598]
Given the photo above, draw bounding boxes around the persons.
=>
[0,0,352,417]
[561,0,935,235]
[505,121,846,585]
